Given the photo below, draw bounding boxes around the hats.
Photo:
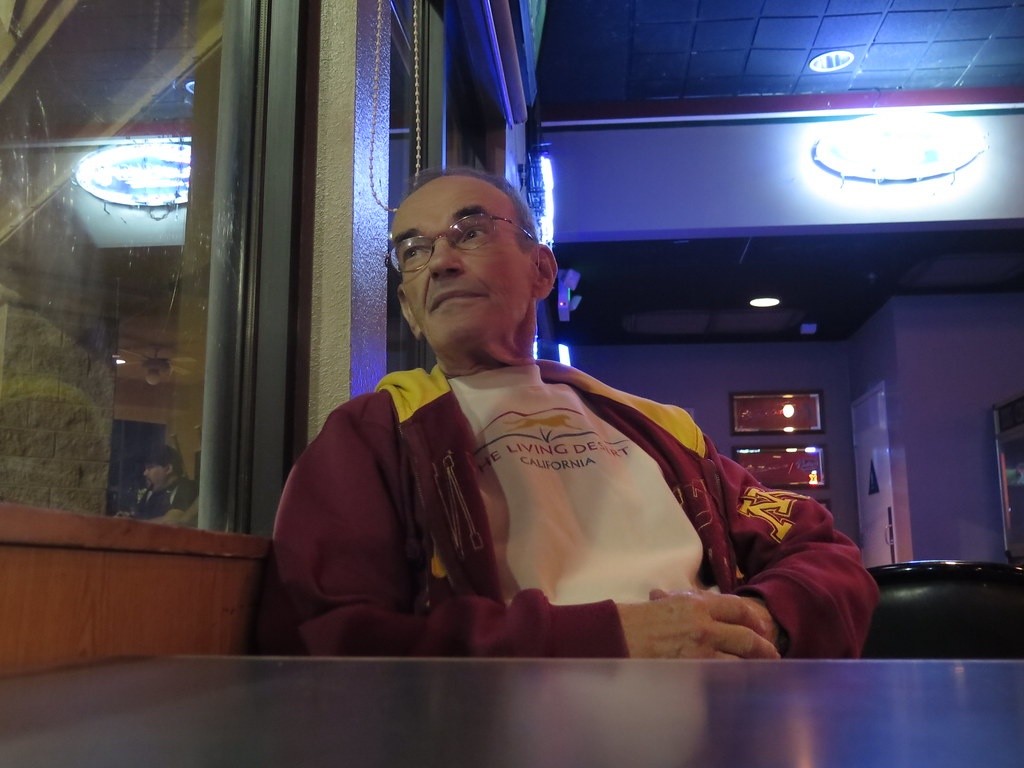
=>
[128,442,183,469]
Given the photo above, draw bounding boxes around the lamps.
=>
[815,111,988,188]
[73,141,191,207]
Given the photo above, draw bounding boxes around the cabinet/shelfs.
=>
[992,388,1024,567]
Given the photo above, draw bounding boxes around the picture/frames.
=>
[731,445,828,489]
[728,389,825,434]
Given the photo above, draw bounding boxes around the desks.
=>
[0,654,1024,768]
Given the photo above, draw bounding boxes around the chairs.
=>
[862,560,1024,661]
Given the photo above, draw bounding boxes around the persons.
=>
[270,167,878,661]
[113,444,198,524]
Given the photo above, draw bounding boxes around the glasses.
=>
[384,213,536,272]
